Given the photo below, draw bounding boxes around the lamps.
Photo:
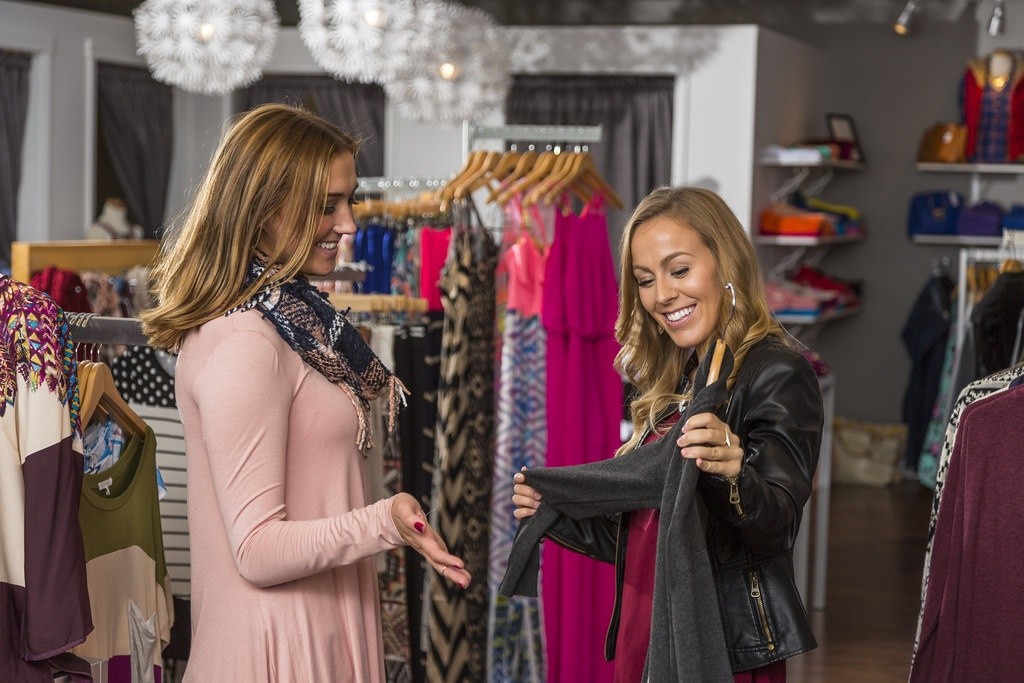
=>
[895,0,925,37]
[985,1,1009,36]
[132,0,520,127]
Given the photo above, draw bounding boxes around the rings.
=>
[441,566,447,576]
[721,427,730,447]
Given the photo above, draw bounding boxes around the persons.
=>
[511,186,824,683]
[956,48,1024,164]
[140,102,472,683]
[87,200,143,240]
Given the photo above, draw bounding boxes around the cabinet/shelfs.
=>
[758,157,870,341]
[910,161,1024,244]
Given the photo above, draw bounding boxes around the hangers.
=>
[64,313,148,442]
[435,124,626,217]
[931,238,1022,301]
[307,262,432,337]
[345,179,490,233]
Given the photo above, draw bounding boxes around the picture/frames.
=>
[823,111,868,164]
